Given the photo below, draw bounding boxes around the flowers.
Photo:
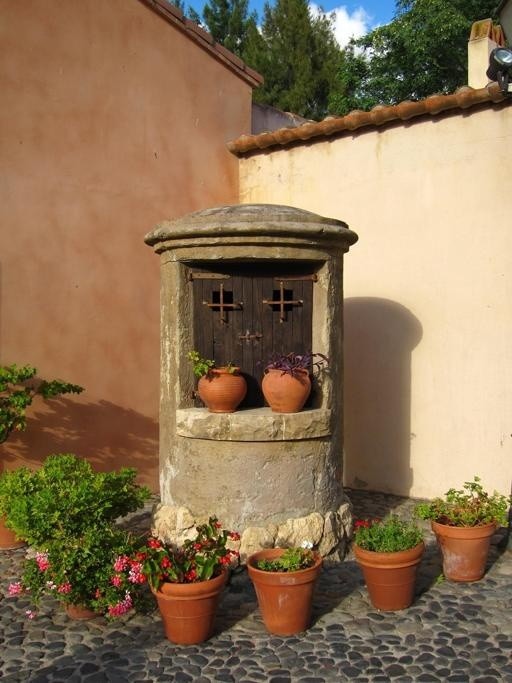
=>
[354,513,423,551]
[252,540,320,572]
[10,528,147,622]
[256,351,333,386]
[111,513,240,586]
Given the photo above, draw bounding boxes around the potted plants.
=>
[186,350,248,413]
[418,476,510,582]
[0,360,150,551]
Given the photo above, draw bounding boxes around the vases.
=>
[352,541,425,610]
[60,596,111,621]
[261,369,311,414]
[150,566,229,646]
[247,547,324,634]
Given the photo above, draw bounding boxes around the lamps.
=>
[485,47,512,96]
